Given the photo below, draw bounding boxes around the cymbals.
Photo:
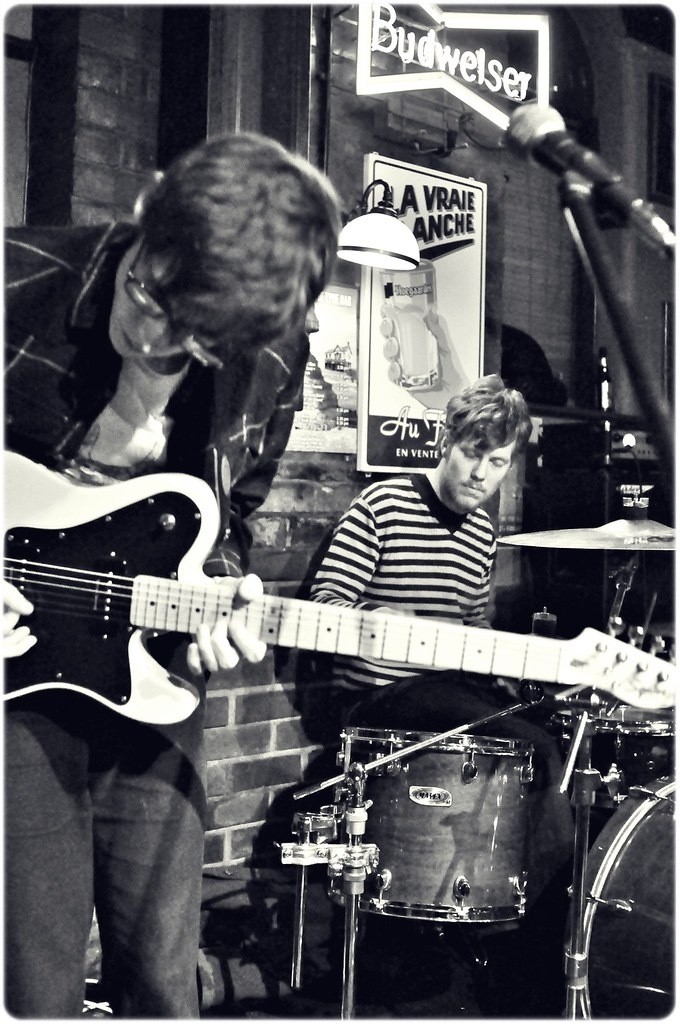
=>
[495,519,675,552]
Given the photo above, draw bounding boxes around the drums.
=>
[326,724,536,922]
[550,706,675,855]
[557,775,676,1020]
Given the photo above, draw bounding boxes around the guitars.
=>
[3,444,677,728]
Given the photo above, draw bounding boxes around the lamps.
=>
[337,178,419,272]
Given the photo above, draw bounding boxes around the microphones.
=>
[503,103,676,261]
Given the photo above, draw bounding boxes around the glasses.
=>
[124,240,237,370]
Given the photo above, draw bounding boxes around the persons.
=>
[4,132,341,1019]
[308,374,574,986]
